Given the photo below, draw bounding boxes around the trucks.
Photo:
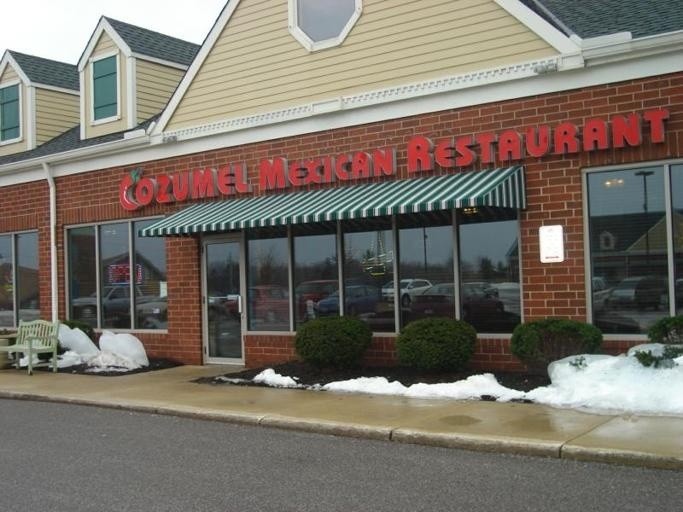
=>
[72,284,216,327]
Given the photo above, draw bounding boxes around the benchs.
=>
[0,319,58,375]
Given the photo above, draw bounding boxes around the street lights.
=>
[634,170,654,250]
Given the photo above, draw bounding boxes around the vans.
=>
[609,274,663,311]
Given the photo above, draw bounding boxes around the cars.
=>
[295,279,339,323]
[382,277,433,308]
[409,281,504,323]
[315,284,381,317]
[220,285,289,323]
